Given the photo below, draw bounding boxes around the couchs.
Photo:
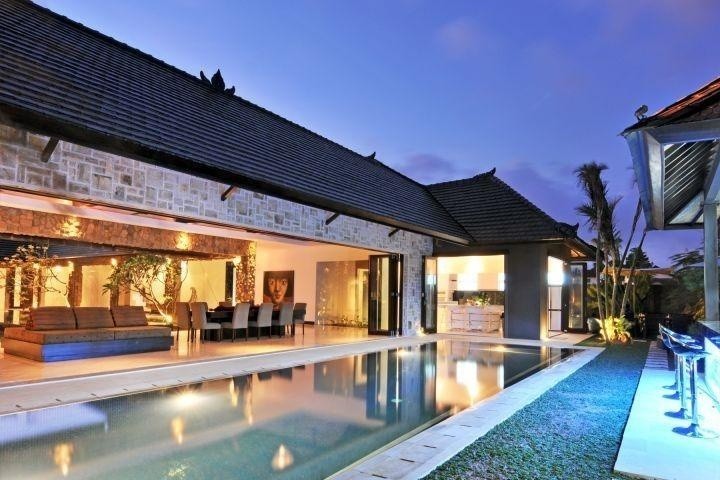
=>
[3,306,174,363]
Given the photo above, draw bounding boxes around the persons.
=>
[263,271,294,311]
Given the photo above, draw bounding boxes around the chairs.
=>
[657,322,717,439]
[175,301,307,344]
